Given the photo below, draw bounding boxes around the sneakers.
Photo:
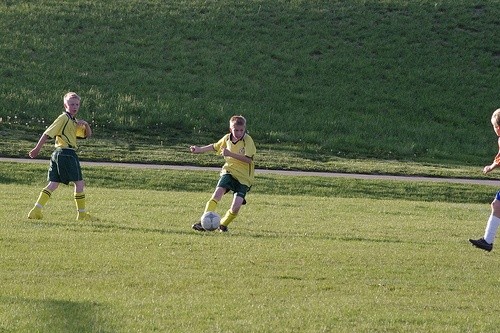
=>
[469,238,494,252]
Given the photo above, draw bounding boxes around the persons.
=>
[190,116,256,231]
[28,92,97,221]
[468,108,500,251]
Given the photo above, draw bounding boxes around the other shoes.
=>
[218,225,228,233]
[192,222,206,231]
[28,210,43,220]
[77,214,96,221]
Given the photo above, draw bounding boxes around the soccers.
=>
[200,211,222,232]
